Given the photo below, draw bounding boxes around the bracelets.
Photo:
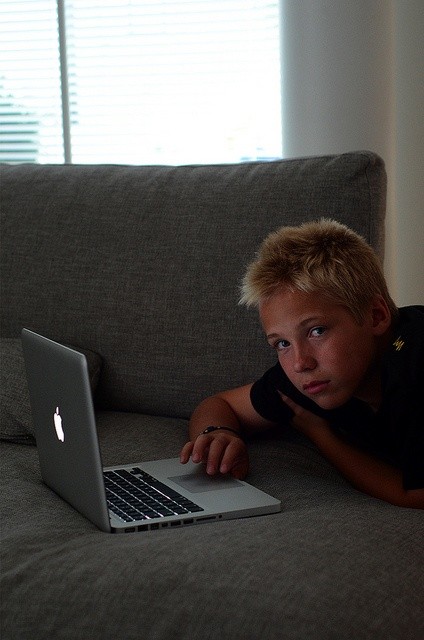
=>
[201,426,239,435]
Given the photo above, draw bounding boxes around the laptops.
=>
[19,326,283,536]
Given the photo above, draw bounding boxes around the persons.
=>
[181,216,423,509]
